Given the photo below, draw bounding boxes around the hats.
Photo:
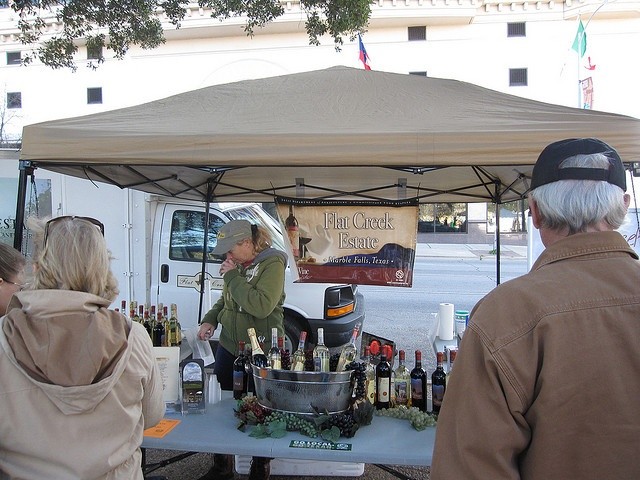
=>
[210,219,254,255]
[529,138,627,192]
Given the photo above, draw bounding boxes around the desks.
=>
[432,335,458,374]
[140,390,438,480]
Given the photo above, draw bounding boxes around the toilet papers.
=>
[429,303,454,343]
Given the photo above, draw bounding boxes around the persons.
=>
[0,242,37,315]
[0,217,165,479]
[197,219,288,392]
[431,138,639,480]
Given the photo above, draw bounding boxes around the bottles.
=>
[361,347,375,410]
[248,326,267,365]
[131,301,139,323]
[338,324,362,374]
[234,339,248,401]
[410,352,428,415]
[138,305,145,328]
[281,203,301,259]
[266,327,280,369]
[450,345,456,371]
[149,305,157,334]
[432,352,446,417]
[277,336,285,350]
[161,306,168,344]
[456,309,468,337]
[395,351,410,408]
[209,373,220,405]
[170,302,182,347]
[289,330,307,372]
[114,305,119,313]
[153,311,165,347]
[313,327,332,376]
[143,311,151,336]
[122,298,128,311]
[376,344,392,412]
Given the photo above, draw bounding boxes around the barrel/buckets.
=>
[250,360,355,423]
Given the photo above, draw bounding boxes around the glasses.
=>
[0,278,35,292]
[43,215,104,249]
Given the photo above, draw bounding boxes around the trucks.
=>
[1,140,366,349]
